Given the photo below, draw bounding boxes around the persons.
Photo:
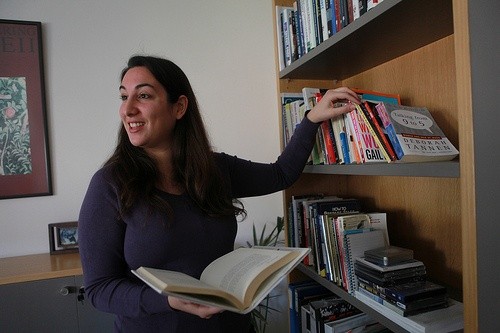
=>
[74,55,363,333]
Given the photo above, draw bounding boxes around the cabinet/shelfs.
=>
[272,0,500,333]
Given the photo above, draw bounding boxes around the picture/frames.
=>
[48,221,79,256]
[0,17,54,200]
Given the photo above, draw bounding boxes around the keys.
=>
[77,288,86,304]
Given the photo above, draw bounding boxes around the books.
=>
[285,189,467,333]
[280,86,462,168]
[128,243,313,316]
[274,0,385,72]
